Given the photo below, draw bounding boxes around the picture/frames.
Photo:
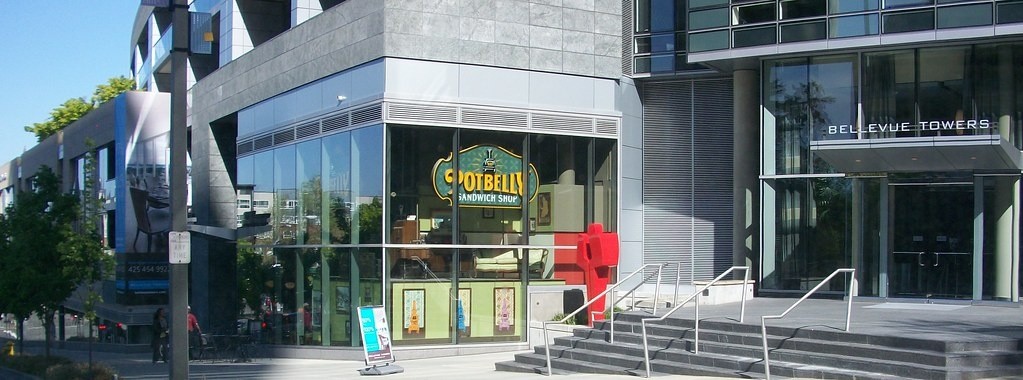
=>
[429,208,460,230]
[483,208,495,219]
[537,192,551,226]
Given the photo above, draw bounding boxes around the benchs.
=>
[472,248,549,278]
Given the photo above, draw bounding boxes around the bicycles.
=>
[157,324,216,364]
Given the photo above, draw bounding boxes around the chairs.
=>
[128,171,170,263]
[199,331,271,363]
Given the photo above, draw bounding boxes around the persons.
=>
[299,303,311,343]
[152,305,203,364]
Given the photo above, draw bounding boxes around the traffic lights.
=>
[98,325,107,330]
[117,322,123,328]
[261,321,272,329]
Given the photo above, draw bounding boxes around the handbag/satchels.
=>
[160,332,166,338]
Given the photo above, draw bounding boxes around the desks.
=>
[211,335,251,363]
